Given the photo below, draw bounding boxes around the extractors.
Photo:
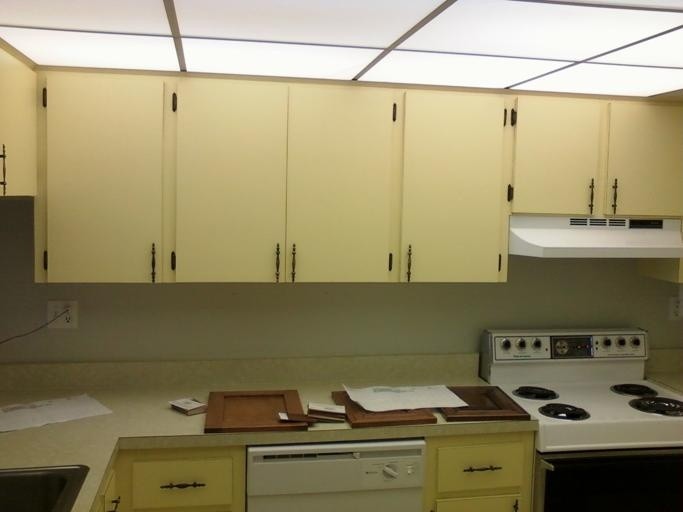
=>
[507,217,683,261]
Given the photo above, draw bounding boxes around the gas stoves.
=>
[496,380,682,454]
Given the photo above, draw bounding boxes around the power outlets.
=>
[46,301,77,329]
[669,297,683,320]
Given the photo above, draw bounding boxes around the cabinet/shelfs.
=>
[34,66,164,284]
[1,36,37,198]
[401,84,512,283]
[424,431,533,512]
[164,72,400,284]
[512,90,683,217]
[93,447,247,512]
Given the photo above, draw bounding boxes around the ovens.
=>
[533,449,683,511]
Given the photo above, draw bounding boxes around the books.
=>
[167,397,207,416]
[278,400,345,423]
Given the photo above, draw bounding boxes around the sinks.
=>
[0,464,90,512]
